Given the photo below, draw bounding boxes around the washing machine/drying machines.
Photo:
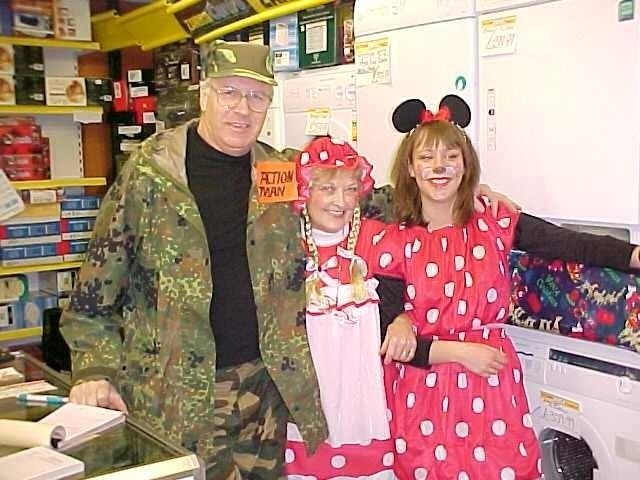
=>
[500,325,640,480]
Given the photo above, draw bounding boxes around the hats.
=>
[203,39,279,86]
[292,136,376,215]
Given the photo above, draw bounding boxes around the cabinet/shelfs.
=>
[0,34,111,342]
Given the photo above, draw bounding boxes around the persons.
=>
[60,38,523,480]
[363,117,640,480]
[284,136,418,479]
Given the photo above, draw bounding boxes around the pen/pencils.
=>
[16,394,67,405]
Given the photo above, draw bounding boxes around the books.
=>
[1,191,105,261]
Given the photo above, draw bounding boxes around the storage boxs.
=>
[8,1,92,40]
[115,42,200,173]
[249,1,355,75]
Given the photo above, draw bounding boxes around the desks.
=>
[0,350,206,478]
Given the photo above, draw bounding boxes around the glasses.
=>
[206,83,272,114]
